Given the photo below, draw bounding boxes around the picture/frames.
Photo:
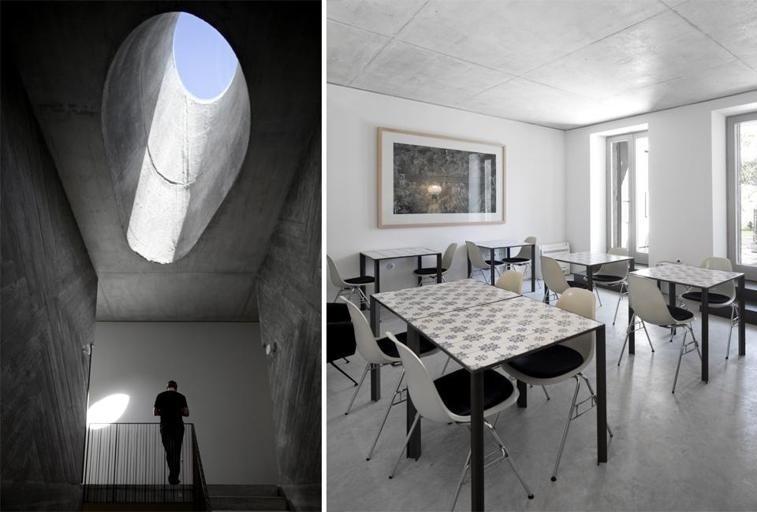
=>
[375,125,507,229]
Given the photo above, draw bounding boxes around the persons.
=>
[152,379,189,485]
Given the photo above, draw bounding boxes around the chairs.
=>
[327,234,757,510]
